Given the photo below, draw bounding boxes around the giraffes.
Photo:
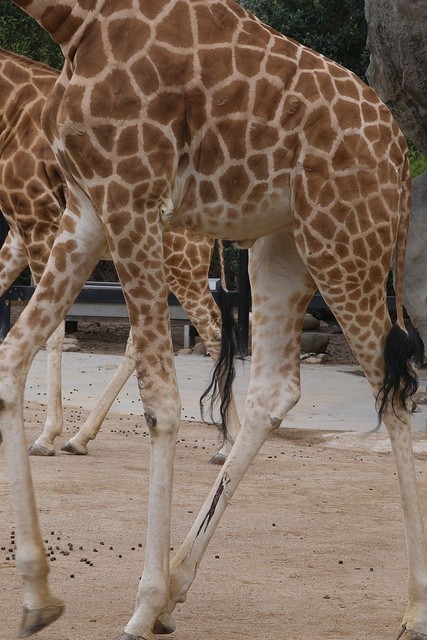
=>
[0,1,427,640]
[1,49,242,465]
